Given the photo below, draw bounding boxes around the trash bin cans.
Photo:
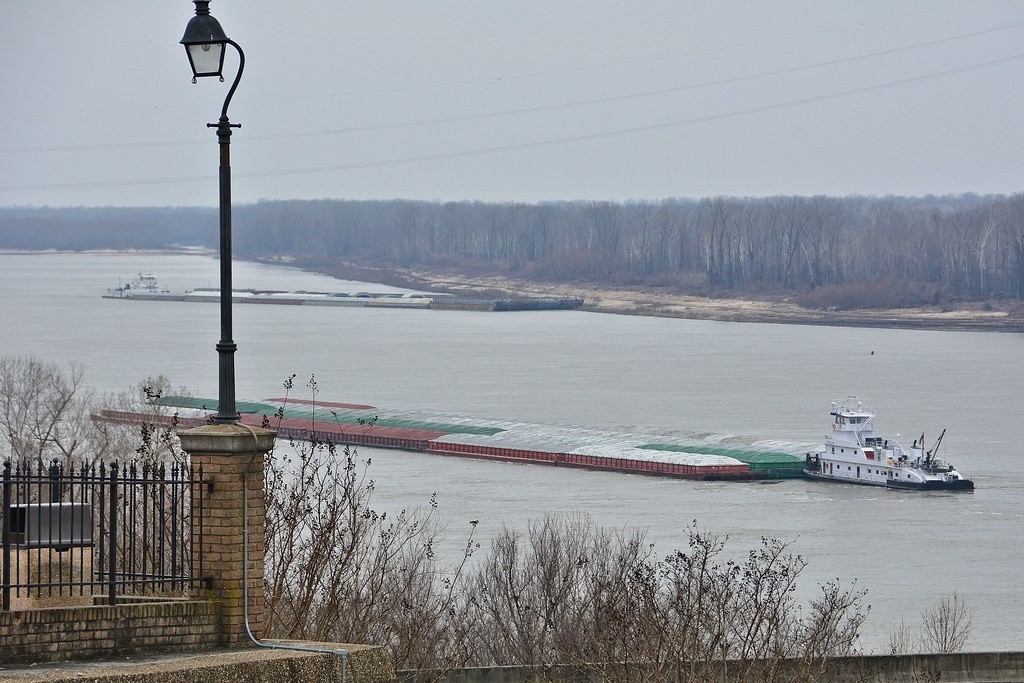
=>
[0,503,95,611]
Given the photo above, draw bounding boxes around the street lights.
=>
[177,0,245,421]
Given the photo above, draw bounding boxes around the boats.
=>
[803,395,976,491]
[102,271,186,302]
